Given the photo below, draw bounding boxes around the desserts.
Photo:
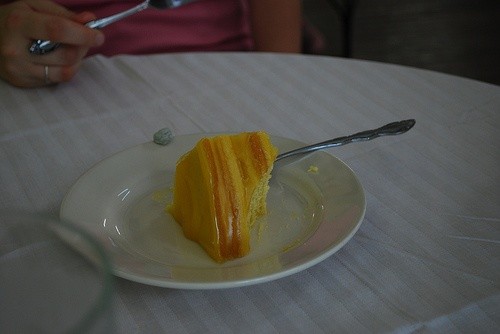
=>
[167,131,277,263]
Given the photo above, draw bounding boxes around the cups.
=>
[1,208,115,334]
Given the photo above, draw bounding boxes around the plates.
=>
[59,130,368,290]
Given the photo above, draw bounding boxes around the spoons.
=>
[27,0,184,56]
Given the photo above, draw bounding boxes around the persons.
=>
[0,0,327,87]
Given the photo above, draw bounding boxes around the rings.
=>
[44,63,51,83]
